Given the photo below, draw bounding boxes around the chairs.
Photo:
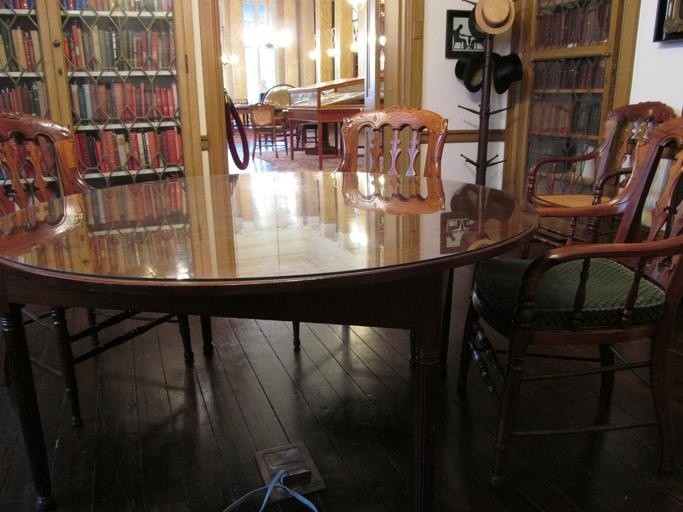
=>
[520,100,682,264]
[1,104,197,430]
[457,112,682,490]
[234,77,365,171]
[288,101,450,356]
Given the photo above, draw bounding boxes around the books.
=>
[0,0,193,270]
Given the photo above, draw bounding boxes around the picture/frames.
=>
[651,0,682,42]
[442,8,494,60]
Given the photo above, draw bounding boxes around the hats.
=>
[455,52,522,94]
[468,0,515,39]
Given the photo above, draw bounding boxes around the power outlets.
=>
[251,438,325,499]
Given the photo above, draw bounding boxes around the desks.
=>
[0,166,543,512]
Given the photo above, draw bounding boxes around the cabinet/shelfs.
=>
[501,0,645,254]
[1,0,212,277]
